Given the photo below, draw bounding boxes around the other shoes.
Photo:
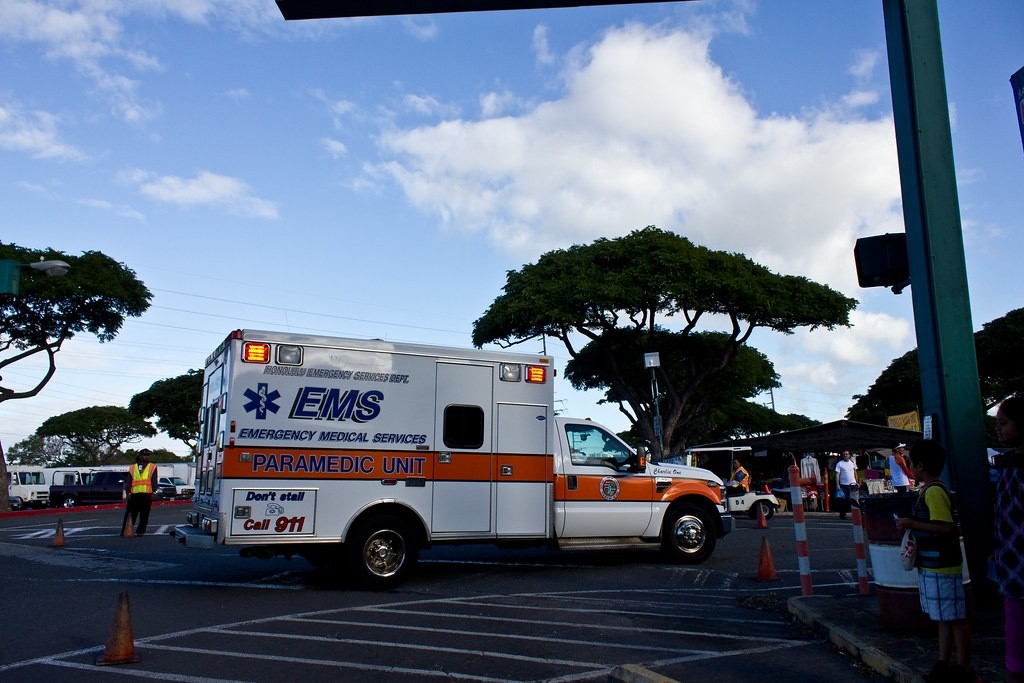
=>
[840,515,846,520]
[927,660,983,682]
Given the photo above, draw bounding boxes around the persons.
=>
[835,449,860,520]
[994,398,1024,682]
[120,449,158,537]
[889,442,916,492]
[895,441,975,682]
[725,458,749,497]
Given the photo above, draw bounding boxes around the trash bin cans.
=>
[859,492,974,634]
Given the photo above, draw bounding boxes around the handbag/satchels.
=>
[900,529,919,570]
[834,488,846,503]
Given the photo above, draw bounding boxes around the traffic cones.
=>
[755,537,779,580]
[95,591,142,666]
[754,503,769,529]
[122,512,136,539]
[49,519,67,547]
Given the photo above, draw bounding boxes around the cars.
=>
[5,464,49,509]
[50,470,146,509]
[154,476,195,497]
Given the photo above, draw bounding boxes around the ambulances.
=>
[189,326,733,594]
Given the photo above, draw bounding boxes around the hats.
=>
[891,441,907,449]
